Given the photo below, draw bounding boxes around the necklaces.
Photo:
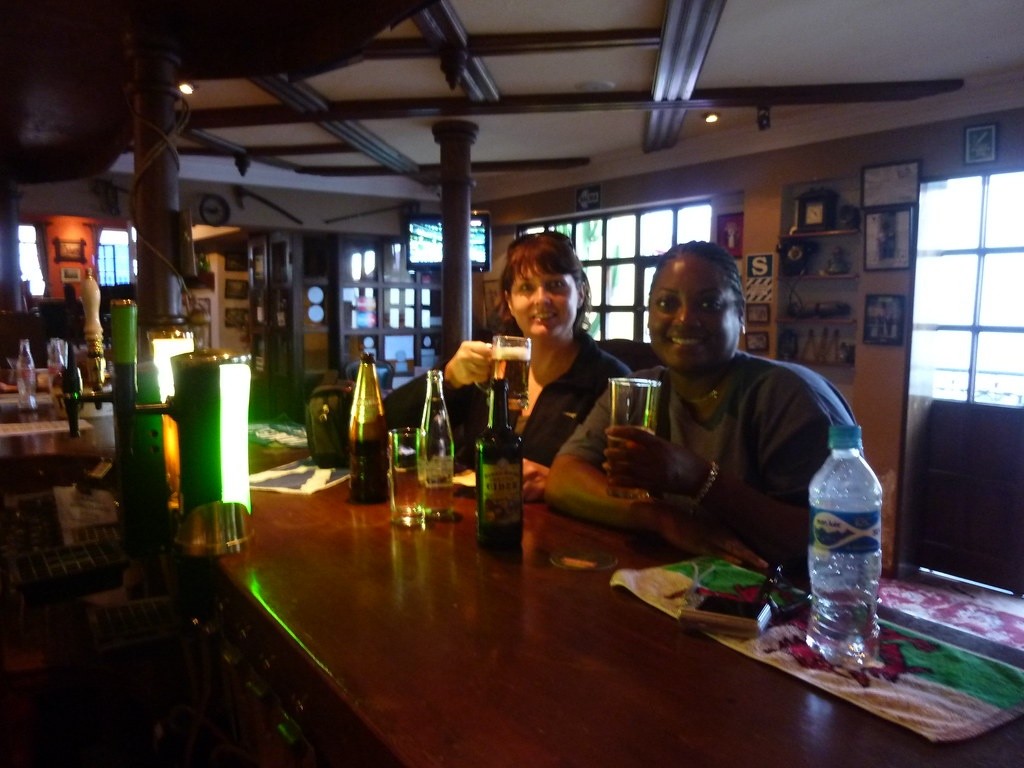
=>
[684,385,719,404]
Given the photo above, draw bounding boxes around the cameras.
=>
[678,594,772,639]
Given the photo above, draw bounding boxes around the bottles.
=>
[349,351,387,507]
[16,339,36,409]
[420,370,455,520]
[47,339,65,387]
[476,381,525,550]
[808,424,882,665]
[803,328,855,368]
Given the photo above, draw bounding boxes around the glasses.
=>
[508,231,574,256]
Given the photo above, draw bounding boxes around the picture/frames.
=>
[859,159,920,209]
[963,123,996,165]
[224,241,248,271]
[862,293,904,346]
[53,239,86,265]
[747,303,770,322]
[862,204,913,271]
[745,331,769,352]
[224,307,247,327]
[61,268,80,281]
[225,279,249,299]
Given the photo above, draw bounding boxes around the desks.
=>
[0,387,1024,768]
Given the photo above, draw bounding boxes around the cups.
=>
[604,377,661,499]
[474,335,532,407]
[777,332,797,362]
[387,428,426,527]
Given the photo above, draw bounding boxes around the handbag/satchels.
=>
[305,382,354,467]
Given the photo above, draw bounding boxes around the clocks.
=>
[199,194,230,226]
[794,188,838,231]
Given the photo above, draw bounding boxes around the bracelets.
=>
[688,458,719,507]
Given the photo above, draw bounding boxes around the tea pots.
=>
[826,257,852,274]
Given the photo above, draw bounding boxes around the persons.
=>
[377,232,636,503]
[544,240,859,571]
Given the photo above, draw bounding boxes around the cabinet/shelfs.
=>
[778,229,860,368]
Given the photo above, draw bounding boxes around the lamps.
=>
[178,82,195,94]
[704,112,719,123]
